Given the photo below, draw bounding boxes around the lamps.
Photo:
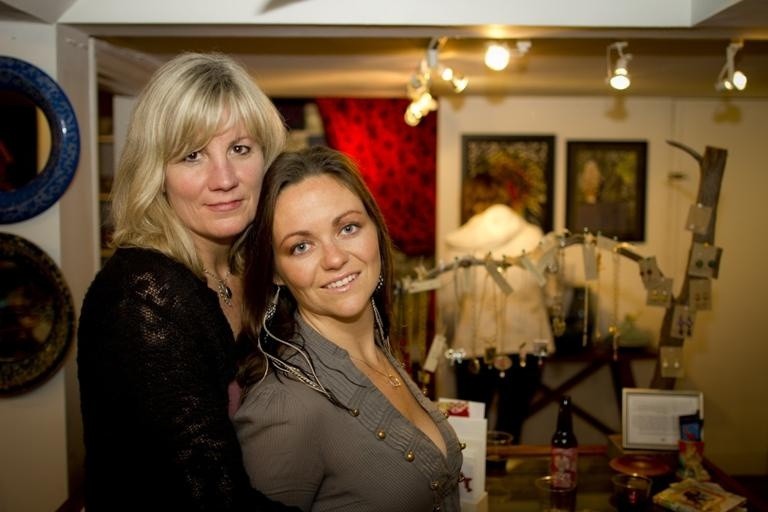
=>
[403,25,469,125]
[481,36,751,98]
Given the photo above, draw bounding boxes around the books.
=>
[607,384,705,460]
[653,478,745,512]
[438,396,487,503]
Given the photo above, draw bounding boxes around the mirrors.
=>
[2,53,82,226]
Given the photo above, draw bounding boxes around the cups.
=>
[533,474,577,512]
[609,474,652,512]
[484,429,512,473]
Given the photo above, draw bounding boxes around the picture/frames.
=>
[565,138,648,241]
[621,387,706,453]
[458,132,557,238]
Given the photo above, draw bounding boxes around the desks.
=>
[479,342,662,444]
[435,442,751,510]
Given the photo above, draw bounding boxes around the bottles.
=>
[550,392,577,507]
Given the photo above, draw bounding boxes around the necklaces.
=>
[194,264,235,306]
[344,347,405,392]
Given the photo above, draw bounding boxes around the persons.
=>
[229,143,461,512]
[434,170,552,474]
[77,50,285,510]
